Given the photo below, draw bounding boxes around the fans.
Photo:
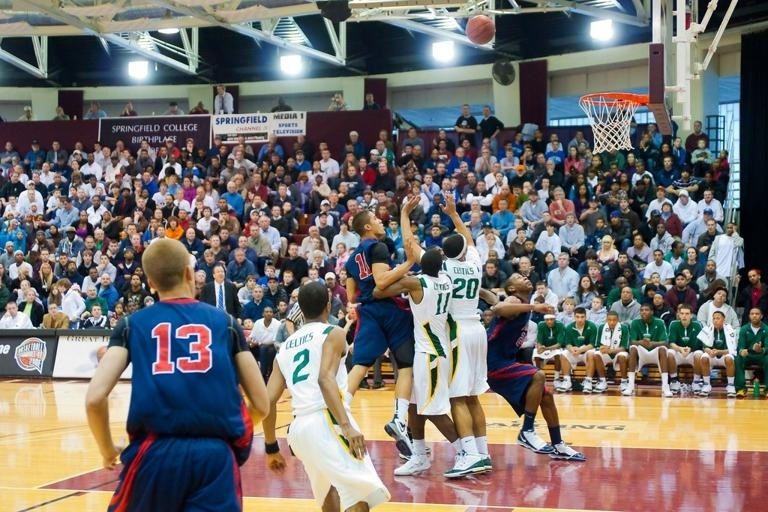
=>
[490,53,518,88]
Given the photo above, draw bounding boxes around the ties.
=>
[218,286,224,311]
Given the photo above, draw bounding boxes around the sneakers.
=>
[554,378,635,395]
[662,381,711,397]
[517,430,586,461]
[385,414,431,475]
[444,455,492,478]
[727,385,747,397]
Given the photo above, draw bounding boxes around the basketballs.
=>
[466,15,495,44]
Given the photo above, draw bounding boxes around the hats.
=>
[704,208,712,214]
[679,190,688,196]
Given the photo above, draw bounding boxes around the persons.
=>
[372,250,466,476]
[337,210,415,456]
[85,240,271,512]
[0,94,767,399]
[486,272,588,461]
[262,284,391,512]
[400,192,493,476]
[213,84,235,114]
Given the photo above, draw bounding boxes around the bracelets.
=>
[264,439,280,454]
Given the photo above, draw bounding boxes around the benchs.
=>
[360,349,762,390]
[271,211,319,271]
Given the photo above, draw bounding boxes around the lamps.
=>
[156,7,180,37]
[314,0,354,22]
